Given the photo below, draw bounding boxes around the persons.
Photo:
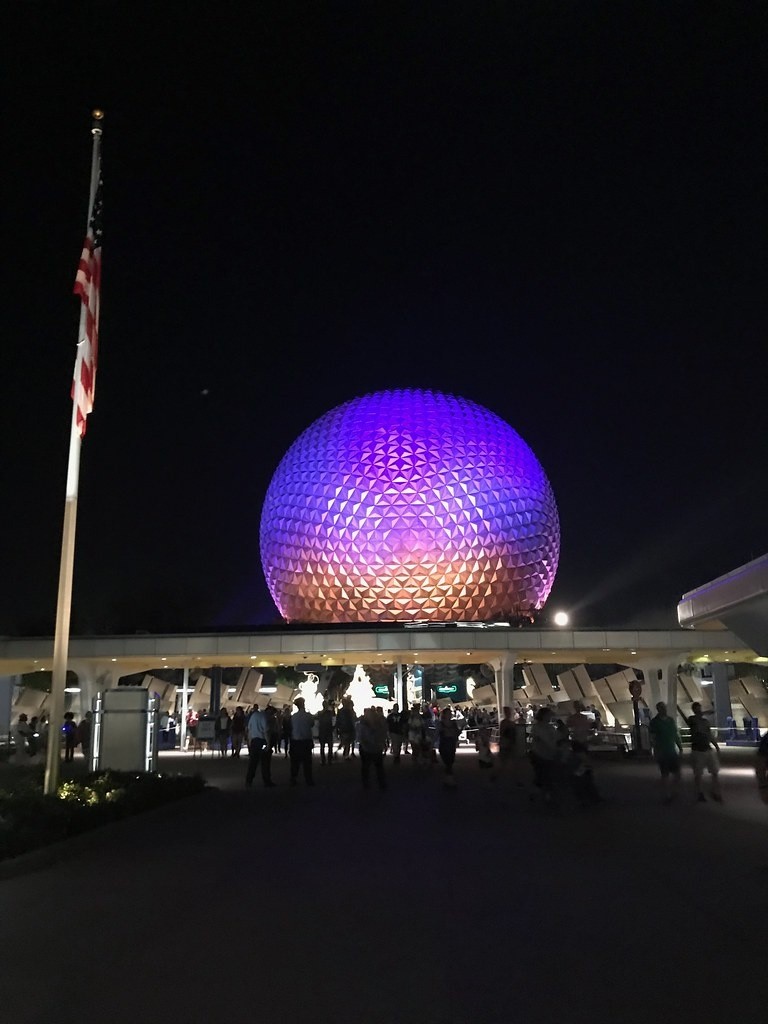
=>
[687,702,723,802]
[160,694,620,808]
[79,711,91,763]
[15,713,49,756]
[63,713,77,764]
[648,702,683,803]
